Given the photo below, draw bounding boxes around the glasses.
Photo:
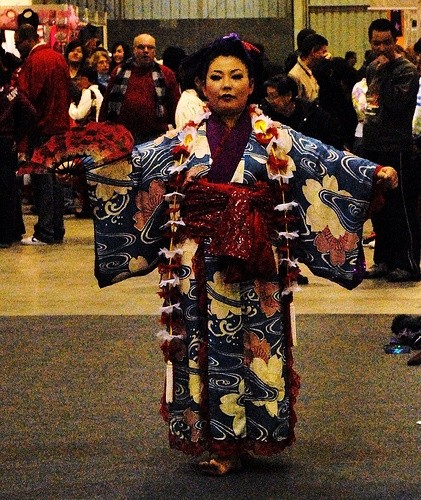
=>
[136,43,156,50]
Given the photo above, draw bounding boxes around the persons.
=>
[0,9,421,282]
[54,34,400,475]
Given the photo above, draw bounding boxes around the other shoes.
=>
[364,263,421,282]
[20,233,47,246]
[197,453,231,477]
